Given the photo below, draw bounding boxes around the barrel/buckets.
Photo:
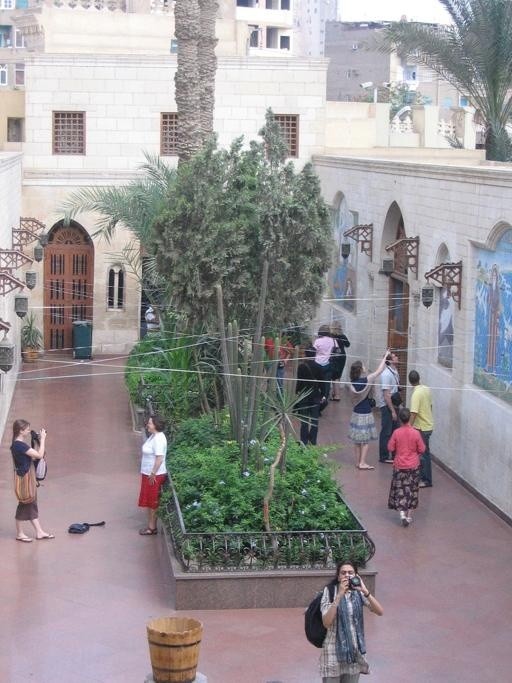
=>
[147,617,201,682]
[24,346,39,362]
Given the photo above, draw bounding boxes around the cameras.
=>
[37,430,47,439]
[345,574,361,590]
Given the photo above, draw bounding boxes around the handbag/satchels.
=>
[15,461,38,504]
[328,347,341,363]
[69,523,89,534]
[391,392,403,407]
[305,584,335,648]
[34,457,47,481]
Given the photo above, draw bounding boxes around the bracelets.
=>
[151,472,155,475]
[364,592,371,597]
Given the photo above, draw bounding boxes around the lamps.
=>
[0,215,48,373]
[338,221,461,310]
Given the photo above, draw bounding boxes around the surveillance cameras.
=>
[360,82,373,88]
[383,82,391,88]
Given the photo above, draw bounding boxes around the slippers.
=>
[36,534,55,539]
[16,537,33,542]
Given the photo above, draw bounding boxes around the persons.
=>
[345,343,393,471]
[407,369,435,488]
[375,349,406,464]
[318,560,384,682]
[137,411,168,535]
[10,417,56,542]
[342,277,355,311]
[264,316,351,446]
[384,407,428,527]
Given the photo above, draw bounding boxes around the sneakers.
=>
[400,510,413,527]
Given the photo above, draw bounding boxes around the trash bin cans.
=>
[73,321,91,359]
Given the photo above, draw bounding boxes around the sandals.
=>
[329,391,340,401]
[139,526,158,535]
